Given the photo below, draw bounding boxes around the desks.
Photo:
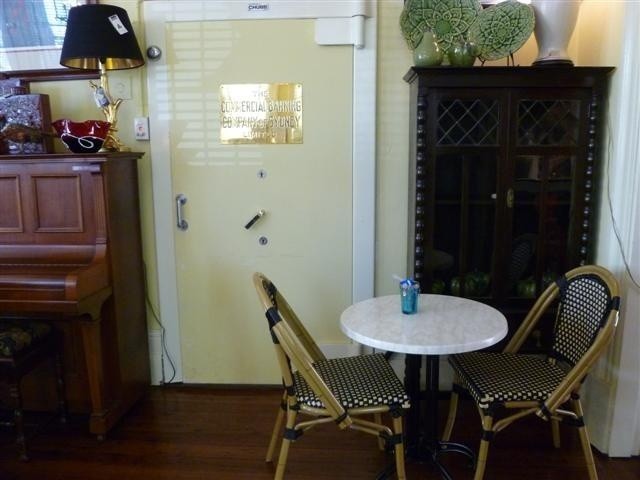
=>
[339,294,509,480]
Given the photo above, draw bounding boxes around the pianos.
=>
[1,152,153,439]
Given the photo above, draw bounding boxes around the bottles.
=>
[412,31,476,67]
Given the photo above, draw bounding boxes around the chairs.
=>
[253,272,413,480]
[442,265,622,479]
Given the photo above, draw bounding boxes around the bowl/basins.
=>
[50,118,111,154]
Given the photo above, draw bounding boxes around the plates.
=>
[401,0,535,61]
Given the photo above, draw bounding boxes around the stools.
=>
[0,319,68,463]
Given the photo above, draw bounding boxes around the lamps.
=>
[60,4,146,152]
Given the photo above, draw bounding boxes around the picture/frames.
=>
[1,0,101,81]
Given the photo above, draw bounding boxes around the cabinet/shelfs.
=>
[403,66,614,423]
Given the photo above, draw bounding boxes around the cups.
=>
[400,278,420,314]
